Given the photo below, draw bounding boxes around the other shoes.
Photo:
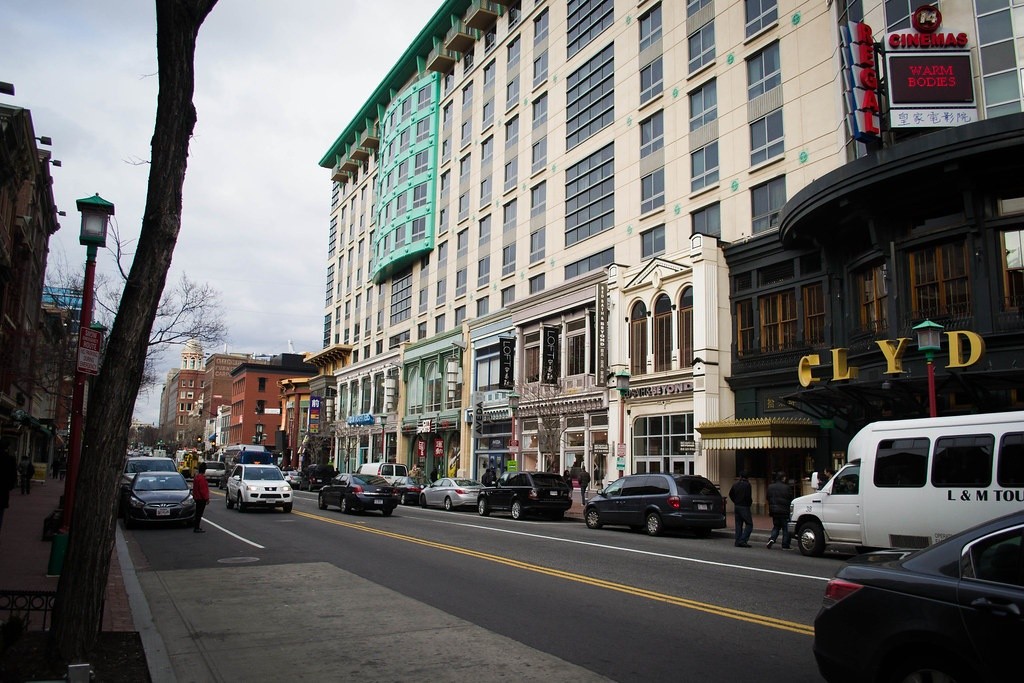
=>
[766,540,774,549]
[783,545,794,551]
[193,528,205,533]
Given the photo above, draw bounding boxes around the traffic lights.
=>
[198,435,201,443]
[212,441,215,447]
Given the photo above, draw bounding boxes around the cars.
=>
[477,472,572,520]
[225,464,293,514]
[122,471,197,530]
[196,460,235,490]
[318,472,402,515]
[391,477,434,504]
[281,464,339,492]
[419,478,486,511]
[121,455,179,515]
[812,508,1024,683]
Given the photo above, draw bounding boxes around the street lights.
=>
[913,318,944,418]
[45,191,115,575]
[380,412,388,459]
[87,322,108,412]
[508,389,521,460]
[252,433,268,446]
[615,369,631,478]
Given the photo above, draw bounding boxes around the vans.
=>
[787,410,1024,557]
[583,474,726,536]
[353,462,409,484]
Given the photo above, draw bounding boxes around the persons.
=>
[767,470,794,551]
[818,466,838,490]
[561,470,573,497]
[482,469,496,487]
[193,462,210,532]
[577,466,590,506]
[0,436,17,545]
[18,455,35,494]
[729,470,753,548]
[50,456,67,481]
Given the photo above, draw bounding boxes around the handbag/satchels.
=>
[26,460,35,480]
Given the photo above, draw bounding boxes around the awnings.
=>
[26,415,52,439]
[695,417,820,450]
